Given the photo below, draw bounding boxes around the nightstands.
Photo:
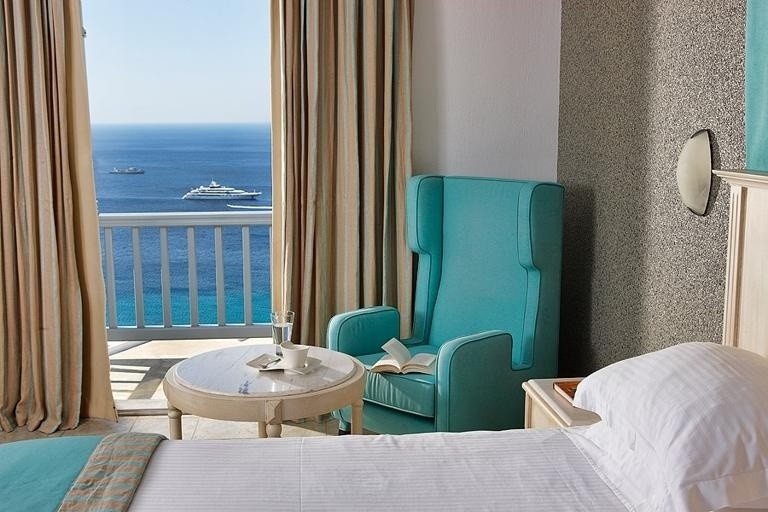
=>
[521,377,601,429]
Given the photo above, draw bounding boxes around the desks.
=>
[164,344,367,439]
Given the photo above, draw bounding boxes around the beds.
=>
[0,168,768,512]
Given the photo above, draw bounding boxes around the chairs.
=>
[326,174,565,435]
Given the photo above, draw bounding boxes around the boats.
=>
[109,167,144,174]
[181,178,263,200]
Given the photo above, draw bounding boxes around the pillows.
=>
[561,422,651,512]
[573,342,768,512]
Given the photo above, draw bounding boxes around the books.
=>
[370,338,438,375]
[552,381,581,408]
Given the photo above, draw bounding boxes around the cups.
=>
[270,310,309,368]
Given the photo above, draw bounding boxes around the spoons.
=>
[263,358,281,369]
